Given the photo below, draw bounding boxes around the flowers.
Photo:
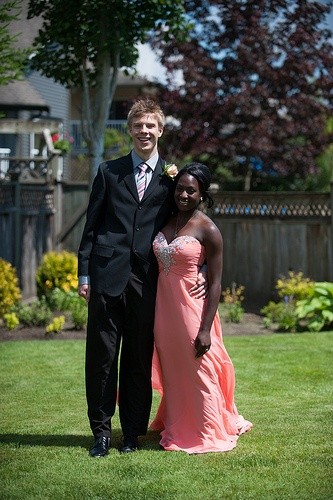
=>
[161,162,179,180]
[50,131,74,152]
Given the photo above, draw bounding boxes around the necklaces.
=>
[173,210,198,238]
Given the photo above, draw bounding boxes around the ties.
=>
[136,162,148,202]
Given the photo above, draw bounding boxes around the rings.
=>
[205,349,208,351]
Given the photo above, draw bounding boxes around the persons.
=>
[117,162,253,454]
[78,98,179,457]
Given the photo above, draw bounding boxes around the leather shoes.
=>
[89,436,110,456]
[120,433,138,452]
